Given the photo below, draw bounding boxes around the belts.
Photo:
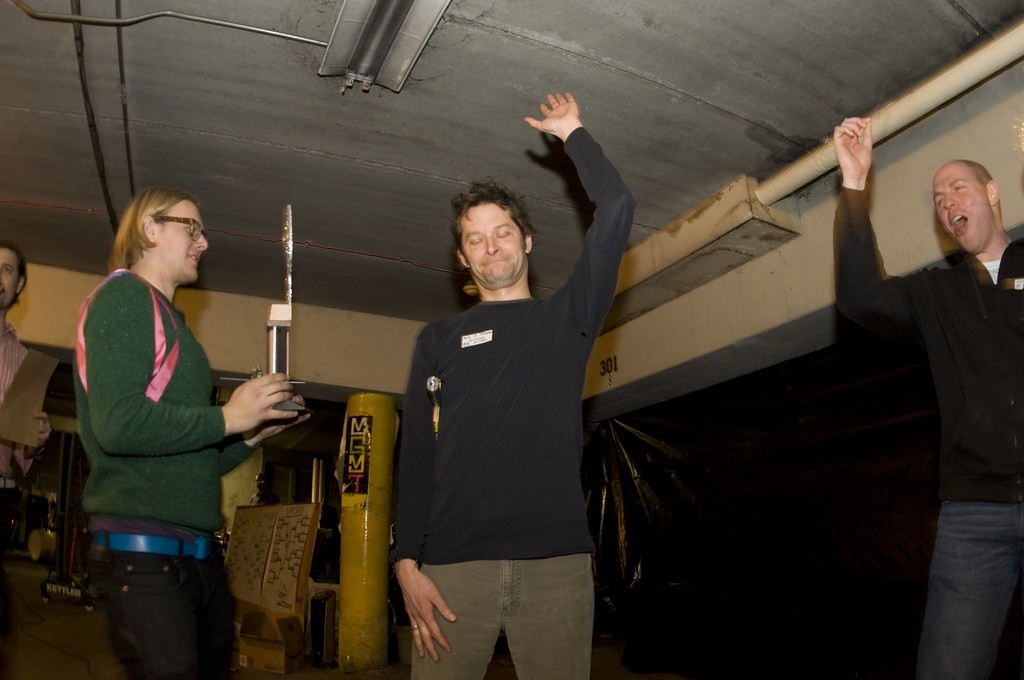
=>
[93,531,213,560]
[0,478,16,488]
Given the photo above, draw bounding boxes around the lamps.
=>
[318,0,453,94]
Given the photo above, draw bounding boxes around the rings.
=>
[409,624,419,631]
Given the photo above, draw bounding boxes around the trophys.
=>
[245,204,306,411]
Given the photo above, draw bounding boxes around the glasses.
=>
[154,216,208,241]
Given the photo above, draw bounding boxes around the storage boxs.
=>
[239,610,305,675]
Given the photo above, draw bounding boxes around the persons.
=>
[249,473,279,505]
[1,241,51,554]
[832,117,1023,680]
[74,186,311,678]
[393,91,633,680]
